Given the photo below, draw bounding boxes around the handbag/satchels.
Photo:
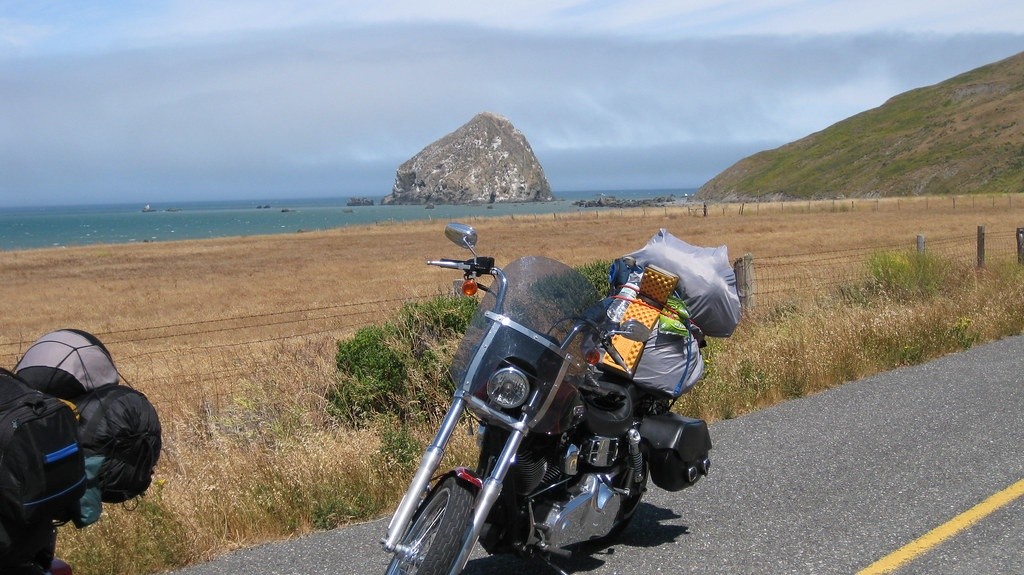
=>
[0,368,86,530]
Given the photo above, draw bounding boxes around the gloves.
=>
[579,386,627,411]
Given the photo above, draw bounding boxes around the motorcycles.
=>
[378,219,714,574]
